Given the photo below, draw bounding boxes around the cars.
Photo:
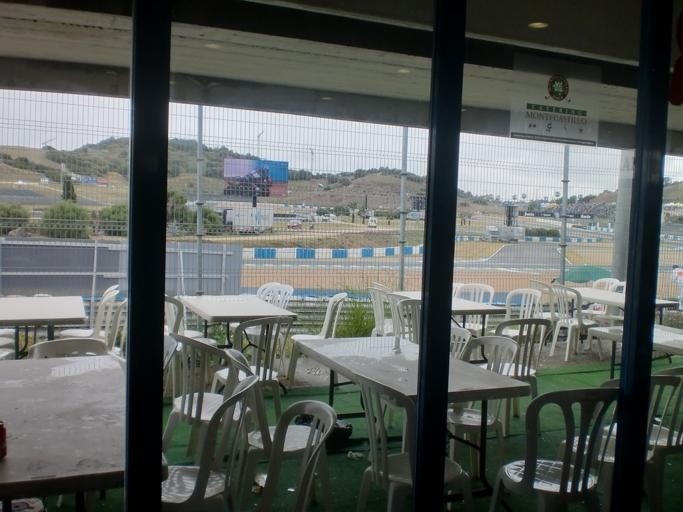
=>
[224,182,270,196]
[287,220,302,229]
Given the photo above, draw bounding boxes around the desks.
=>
[588,322,683,377]
[392,290,507,349]
[179,295,297,391]
[0,355,169,512]
[0,295,87,342]
[542,285,679,350]
[297,336,531,452]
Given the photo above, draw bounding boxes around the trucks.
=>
[232,207,273,234]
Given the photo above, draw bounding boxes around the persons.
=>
[244,166,269,196]
[671,265,683,310]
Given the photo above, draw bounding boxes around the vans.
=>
[368,217,377,228]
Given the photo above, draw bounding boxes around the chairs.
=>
[368,287,412,336]
[489,288,542,363]
[597,282,624,326]
[470,318,551,437]
[357,375,472,512]
[168,302,204,338]
[373,281,411,328]
[255,399,337,512]
[384,325,471,451]
[453,282,461,295]
[0,348,16,360]
[163,333,177,395]
[54,290,120,338]
[166,296,217,393]
[531,280,572,352]
[454,284,494,361]
[224,349,328,512]
[160,375,260,512]
[287,293,347,379]
[221,281,280,366]
[26,338,108,358]
[232,284,294,378]
[603,367,683,512]
[0,337,17,348]
[163,332,252,472]
[388,295,412,334]
[60,284,118,344]
[300,298,345,389]
[0,328,16,336]
[551,285,604,362]
[447,336,519,507]
[211,316,293,425]
[574,277,620,350]
[559,376,683,512]
[489,388,620,511]
[70,298,128,356]
